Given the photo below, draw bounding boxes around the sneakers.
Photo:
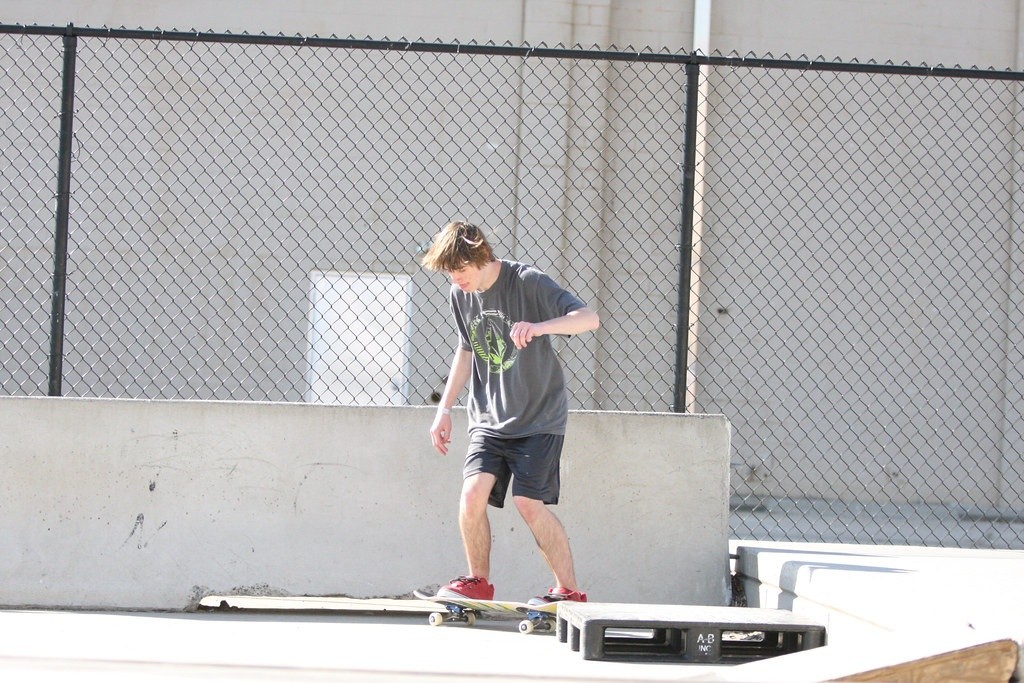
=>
[437,575,494,600]
[527,587,587,606]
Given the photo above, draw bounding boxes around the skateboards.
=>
[411,586,573,635]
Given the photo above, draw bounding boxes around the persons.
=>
[423,222,600,606]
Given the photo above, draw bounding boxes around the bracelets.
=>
[437,408,451,416]
